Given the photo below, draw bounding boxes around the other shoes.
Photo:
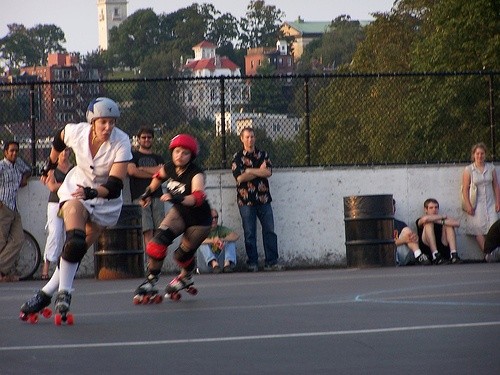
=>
[0,274,19,281]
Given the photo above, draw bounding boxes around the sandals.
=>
[41,274,49,279]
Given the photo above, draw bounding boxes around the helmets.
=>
[86,97,120,124]
[168,133,199,155]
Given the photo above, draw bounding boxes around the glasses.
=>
[140,136,153,139]
[212,216,218,219]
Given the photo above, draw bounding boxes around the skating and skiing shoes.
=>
[19,289,53,324]
[164,271,198,301]
[133,264,162,305]
[54,290,74,326]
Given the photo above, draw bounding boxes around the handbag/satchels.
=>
[461,166,477,213]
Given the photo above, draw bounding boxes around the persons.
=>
[416,198,463,265]
[127,126,167,276]
[462,142,500,263]
[484,218,500,263]
[393,198,433,266]
[133,134,213,295]
[197,208,240,274]
[0,141,32,282]
[41,147,75,280]
[19,96,133,314]
[231,128,287,272]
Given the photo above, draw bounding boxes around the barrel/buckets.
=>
[343,194,396,268]
[94,205,145,281]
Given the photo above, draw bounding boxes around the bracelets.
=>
[442,219,445,225]
[441,215,444,220]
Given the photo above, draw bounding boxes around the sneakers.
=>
[213,265,221,273]
[264,262,286,271]
[433,253,445,265]
[450,253,465,264]
[415,253,431,265]
[223,266,233,273]
[247,265,258,272]
